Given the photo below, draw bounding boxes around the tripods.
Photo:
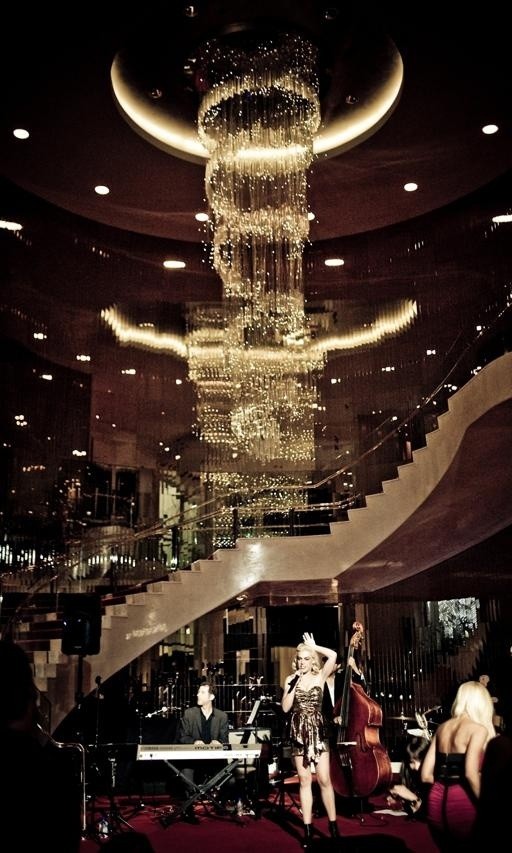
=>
[80,691,107,850]
[266,743,304,818]
[89,754,135,834]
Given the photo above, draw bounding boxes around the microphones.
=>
[291,669,303,685]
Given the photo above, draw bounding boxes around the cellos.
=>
[329,622,392,798]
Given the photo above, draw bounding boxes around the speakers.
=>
[62,592,101,655]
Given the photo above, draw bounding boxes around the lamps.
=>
[184,19,325,482]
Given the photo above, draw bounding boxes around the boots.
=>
[302,824,313,848]
[328,821,340,837]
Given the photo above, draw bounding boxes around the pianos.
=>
[136,742,262,760]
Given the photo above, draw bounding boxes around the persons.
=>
[0,641,82,853]
[322,656,369,819]
[420,681,497,853]
[282,631,343,849]
[177,680,235,816]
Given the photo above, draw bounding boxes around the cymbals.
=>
[389,716,414,721]
